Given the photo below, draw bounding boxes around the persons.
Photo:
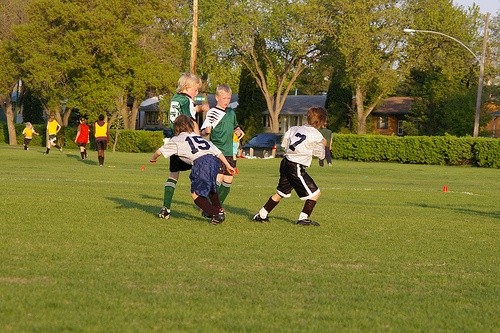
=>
[232,128,244,160]
[319,122,333,167]
[93,114,109,166]
[252,106,327,225]
[150,114,235,224]
[158,74,207,221]
[46,115,63,154]
[75,117,90,159]
[22,122,39,151]
[237,136,244,158]
[201,84,240,217]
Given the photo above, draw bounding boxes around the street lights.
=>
[403,13,492,137]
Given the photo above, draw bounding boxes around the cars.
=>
[240,132,285,158]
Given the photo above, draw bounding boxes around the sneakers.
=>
[157,205,171,219]
[202,210,212,219]
[210,212,225,225]
[85,154,88,159]
[99,164,103,166]
[328,163,332,167]
[296,219,320,227]
[252,212,271,223]
[59,146,62,153]
